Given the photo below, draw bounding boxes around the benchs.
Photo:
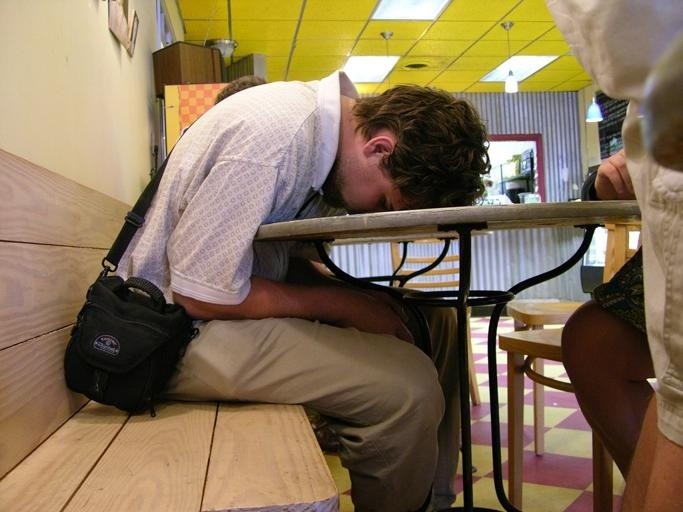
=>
[0,150,340,512]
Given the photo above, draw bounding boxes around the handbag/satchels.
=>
[63,274,193,416]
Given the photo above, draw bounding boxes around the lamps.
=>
[585,79,604,122]
[205,39,238,58]
[380,33,394,88]
[500,21,518,93]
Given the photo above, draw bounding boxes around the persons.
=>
[104,68,494,512]
[215,75,268,106]
[543,0,683,512]
[560,147,657,481]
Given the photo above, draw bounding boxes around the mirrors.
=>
[472,134,544,206]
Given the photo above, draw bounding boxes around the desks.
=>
[253,200,641,511]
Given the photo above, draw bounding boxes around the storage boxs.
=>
[153,41,221,98]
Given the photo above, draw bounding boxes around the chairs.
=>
[499,222,643,512]
[506,225,614,456]
[391,239,481,406]
